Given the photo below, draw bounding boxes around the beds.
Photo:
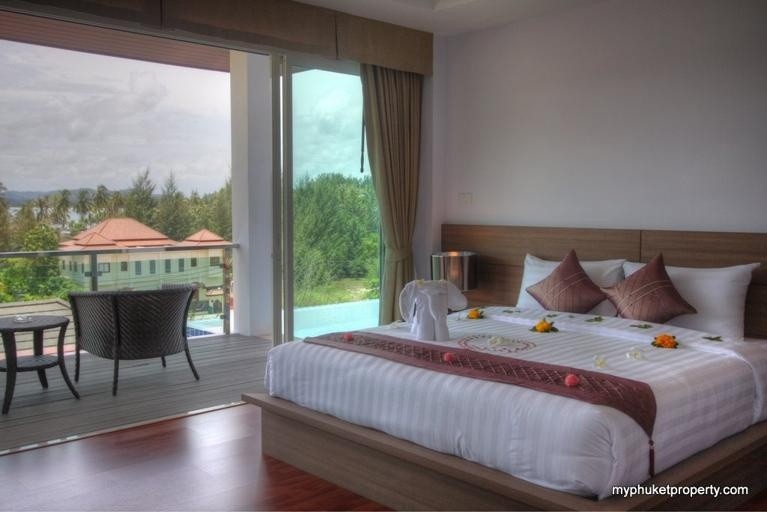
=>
[242,304,766,511]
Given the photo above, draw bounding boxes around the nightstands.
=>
[447,290,497,314]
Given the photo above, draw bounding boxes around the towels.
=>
[400,278,468,342]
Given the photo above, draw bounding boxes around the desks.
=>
[0,316,80,416]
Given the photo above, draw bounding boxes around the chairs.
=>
[67,282,199,398]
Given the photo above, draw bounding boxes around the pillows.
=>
[525,248,606,314]
[600,252,697,324]
[618,261,762,343]
[515,253,625,316]
